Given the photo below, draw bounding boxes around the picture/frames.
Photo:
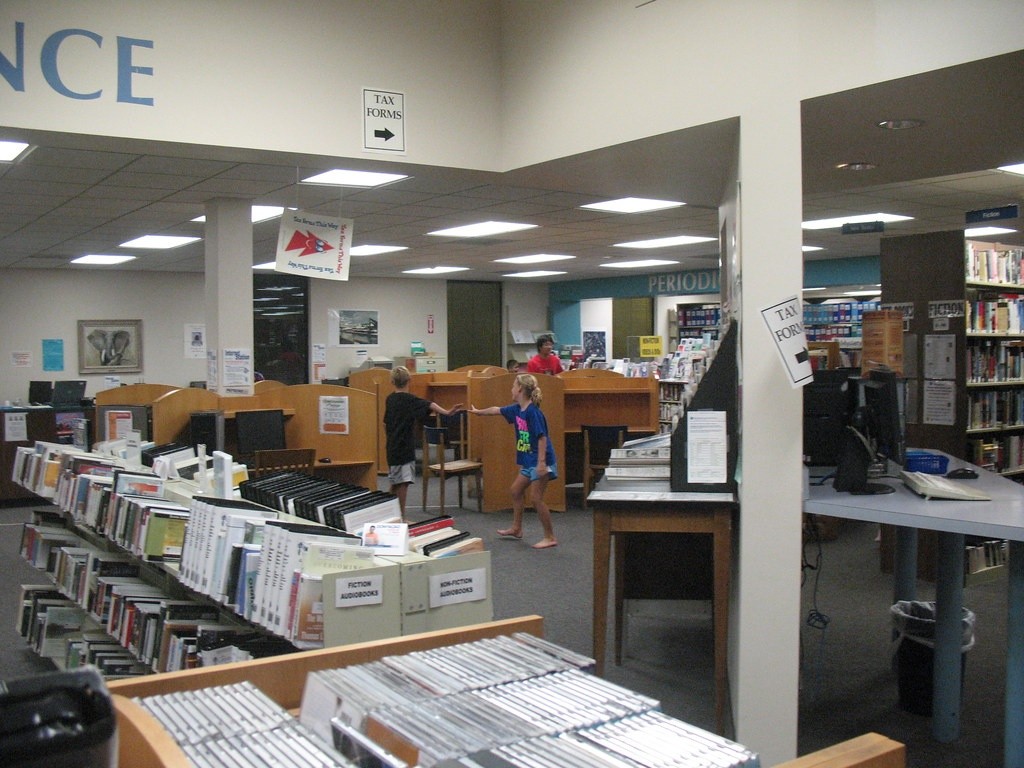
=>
[78,319,143,374]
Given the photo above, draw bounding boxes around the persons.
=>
[381,366,464,525]
[506,359,519,373]
[466,373,561,549]
[272,360,297,386]
[526,334,564,374]
[277,337,307,383]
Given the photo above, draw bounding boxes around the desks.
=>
[0,364,1024,768]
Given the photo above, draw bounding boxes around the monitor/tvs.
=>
[803,364,904,467]
[236,409,286,453]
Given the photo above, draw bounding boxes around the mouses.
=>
[319,458,331,463]
[946,468,979,479]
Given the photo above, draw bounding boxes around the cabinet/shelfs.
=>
[876,229,1023,479]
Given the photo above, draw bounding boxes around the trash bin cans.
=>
[889,600,974,718]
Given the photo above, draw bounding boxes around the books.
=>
[3,417,762,768]
[563,241,1024,502]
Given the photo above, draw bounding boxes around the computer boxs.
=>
[190,409,225,456]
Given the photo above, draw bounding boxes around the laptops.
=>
[29,381,86,410]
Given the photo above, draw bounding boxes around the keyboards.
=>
[898,471,990,502]
[233,455,256,469]
